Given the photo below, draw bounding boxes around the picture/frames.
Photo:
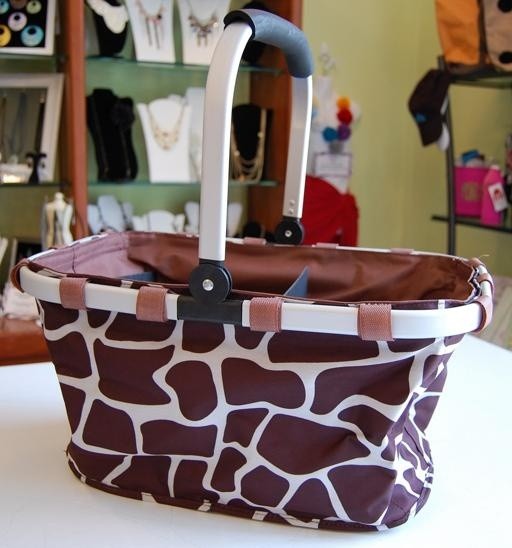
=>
[0,73,65,183]
[0,0,57,56]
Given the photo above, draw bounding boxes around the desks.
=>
[0,334,512,548]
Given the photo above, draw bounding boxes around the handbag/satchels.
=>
[11,9,495,531]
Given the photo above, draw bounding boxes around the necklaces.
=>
[135,2,169,51]
[145,209,178,235]
[90,94,132,184]
[185,1,224,49]
[230,106,268,186]
[89,1,128,35]
[147,100,187,151]
[98,198,130,234]
[312,88,354,147]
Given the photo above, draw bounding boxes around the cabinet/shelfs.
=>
[0,0,303,366]
[429,56,512,255]
